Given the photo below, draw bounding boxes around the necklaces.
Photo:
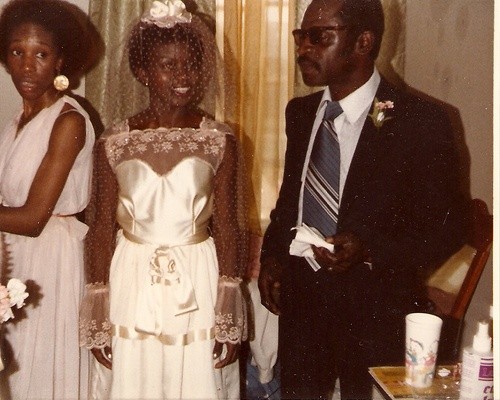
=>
[15,90,60,128]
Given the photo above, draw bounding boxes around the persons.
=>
[257,2,469,397]
[0,0,94,397]
[84,0,252,397]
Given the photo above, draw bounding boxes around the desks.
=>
[368,365,462,400]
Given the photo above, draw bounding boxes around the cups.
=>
[405,313,443,387]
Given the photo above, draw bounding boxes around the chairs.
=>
[428,198,493,365]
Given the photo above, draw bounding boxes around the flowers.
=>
[0,278,30,324]
[368,97,395,132]
[139,0,193,30]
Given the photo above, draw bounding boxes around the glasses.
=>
[292,24,353,46]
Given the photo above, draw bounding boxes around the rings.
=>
[328,267,334,273]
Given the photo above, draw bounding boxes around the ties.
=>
[302,102,343,273]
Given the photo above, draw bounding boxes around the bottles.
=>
[459,322,494,400]
